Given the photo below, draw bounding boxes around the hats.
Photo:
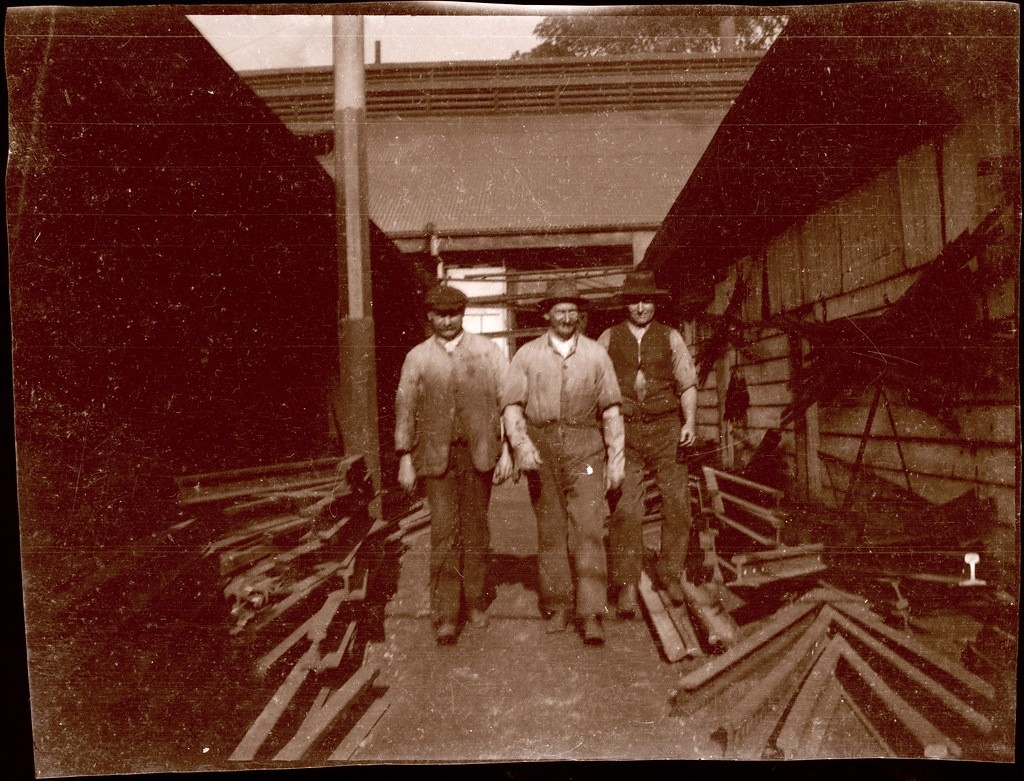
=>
[611,271,670,300]
[422,286,468,311]
[533,280,591,309]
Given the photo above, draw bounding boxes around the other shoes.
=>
[582,615,606,645]
[615,584,639,619]
[434,622,459,642]
[666,579,684,607]
[544,608,572,634]
[466,608,491,629]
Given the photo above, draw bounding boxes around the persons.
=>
[596,268,698,619]
[496,280,626,645]
[392,285,514,646]
